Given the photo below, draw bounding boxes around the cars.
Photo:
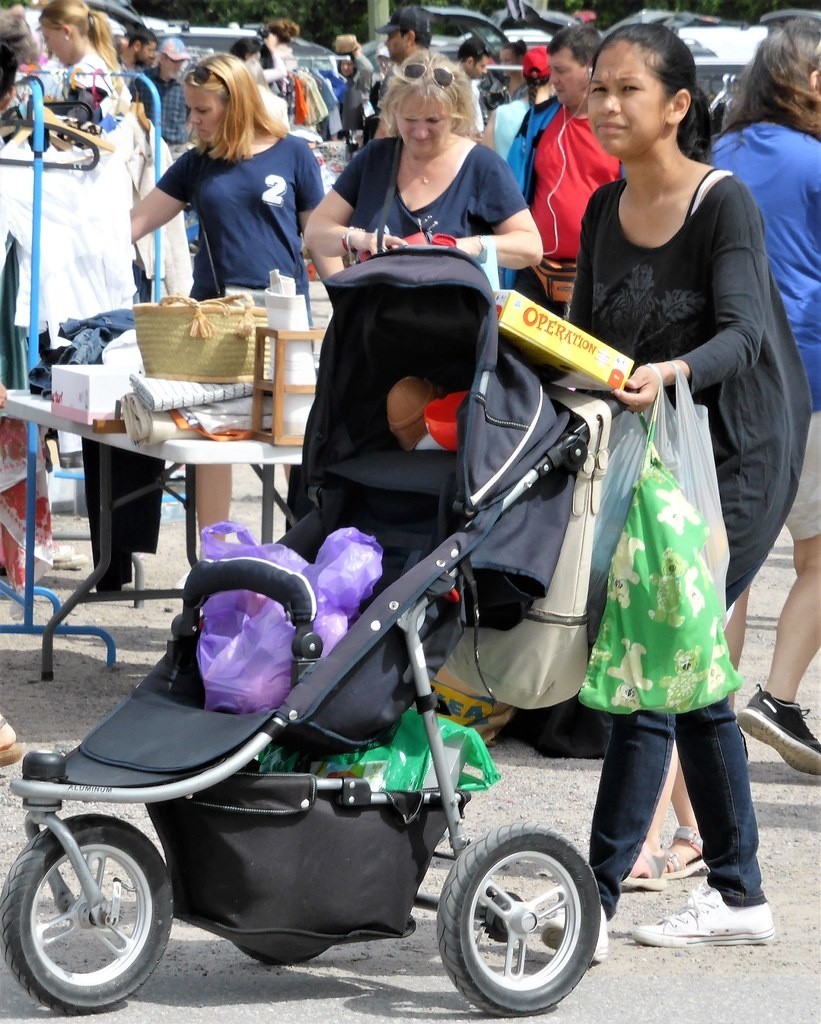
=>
[110,8,768,118]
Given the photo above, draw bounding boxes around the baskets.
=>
[131,296,272,384]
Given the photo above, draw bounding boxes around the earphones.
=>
[588,66,593,77]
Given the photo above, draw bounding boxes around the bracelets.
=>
[476,235,488,264]
[341,226,364,265]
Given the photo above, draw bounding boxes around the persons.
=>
[0,0,708,891]
[303,51,542,270]
[704,14,821,774]
[542,25,812,968]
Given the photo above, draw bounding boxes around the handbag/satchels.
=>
[264,704,500,797]
[566,357,743,716]
[194,520,382,715]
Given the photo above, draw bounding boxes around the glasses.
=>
[473,45,491,56]
[185,67,219,84]
[402,65,456,89]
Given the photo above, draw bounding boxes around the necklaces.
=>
[403,151,441,185]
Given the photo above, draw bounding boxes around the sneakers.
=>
[736,684,821,774]
[631,876,778,946]
[543,902,609,965]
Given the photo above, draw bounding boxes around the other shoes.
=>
[53,548,89,568]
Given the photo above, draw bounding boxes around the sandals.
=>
[0,716,22,766]
[660,827,709,880]
[619,843,666,891]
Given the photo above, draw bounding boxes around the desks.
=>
[4,390,304,682]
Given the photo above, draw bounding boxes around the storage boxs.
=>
[424,731,473,791]
[51,363,132,423]
[493,289,635,391]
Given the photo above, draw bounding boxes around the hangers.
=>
[0,68,150,170]
[285,57,315,72]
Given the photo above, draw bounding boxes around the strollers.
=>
[0,244,654,1019]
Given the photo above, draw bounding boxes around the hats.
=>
[160,37,192,61]
[374,5,432,37]
[521,45,551,77]
[374,42,390,60]
